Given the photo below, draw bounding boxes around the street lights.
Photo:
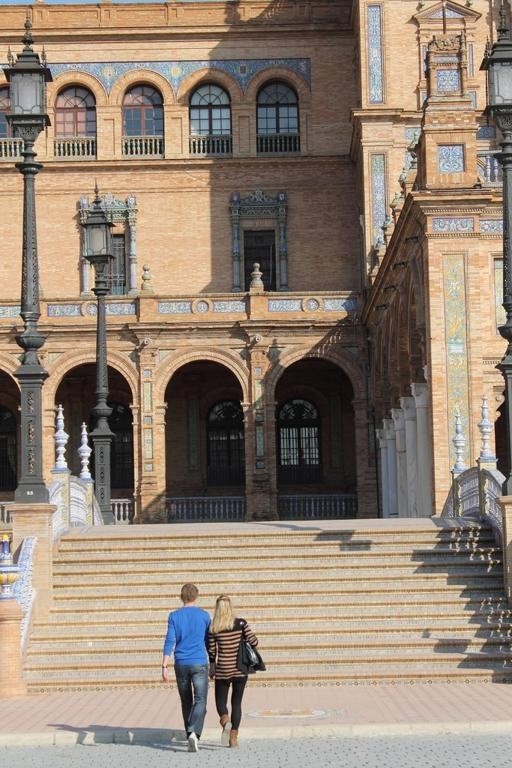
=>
[479,6,510,497]
[79,180,116,528]
[2,13,52,505]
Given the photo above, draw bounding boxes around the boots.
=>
[220,714,238,748]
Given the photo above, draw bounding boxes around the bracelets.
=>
[162,663,167,668]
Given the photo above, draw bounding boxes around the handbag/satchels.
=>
[237,640,266,673]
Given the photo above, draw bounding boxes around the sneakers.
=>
[187,732,198,752]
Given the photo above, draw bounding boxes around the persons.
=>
[204,595,260,749]
[161,583,214,753]
